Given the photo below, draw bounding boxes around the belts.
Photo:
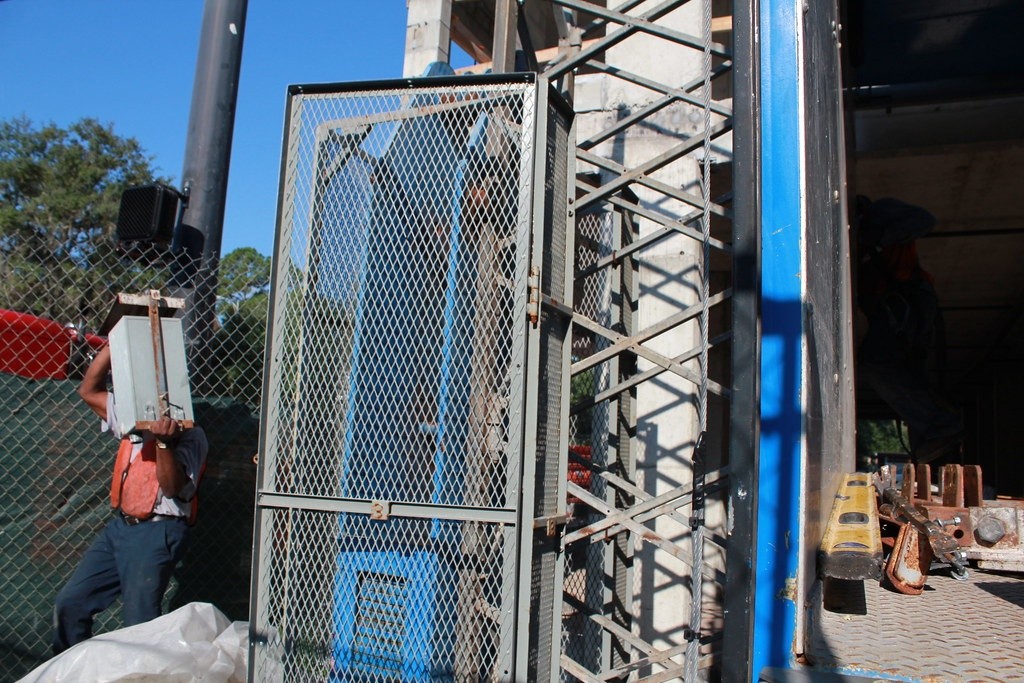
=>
[120,512,179,526]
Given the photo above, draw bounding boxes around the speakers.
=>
[115,183,179,244]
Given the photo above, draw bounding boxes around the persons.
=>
[49,342,211,657]
